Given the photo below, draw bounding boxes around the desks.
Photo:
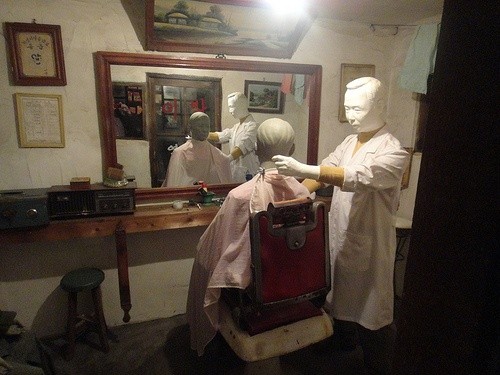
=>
[0,197,224,248]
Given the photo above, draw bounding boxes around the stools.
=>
[54,267,119,359]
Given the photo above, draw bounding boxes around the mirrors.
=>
[95,52,327,202]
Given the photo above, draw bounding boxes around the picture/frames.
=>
[16,92,67,149]
[3,21,68,87]
[146,0,321,60]
[244,80,287,114]
[339,64,377,123]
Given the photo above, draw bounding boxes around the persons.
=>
[273,76,411,375]
[166,112,250,188]
[187,118,316,357]
[208,92,260,170]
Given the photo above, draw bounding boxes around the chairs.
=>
[223,200,335,375]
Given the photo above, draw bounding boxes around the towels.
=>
[397,23,439,95]
